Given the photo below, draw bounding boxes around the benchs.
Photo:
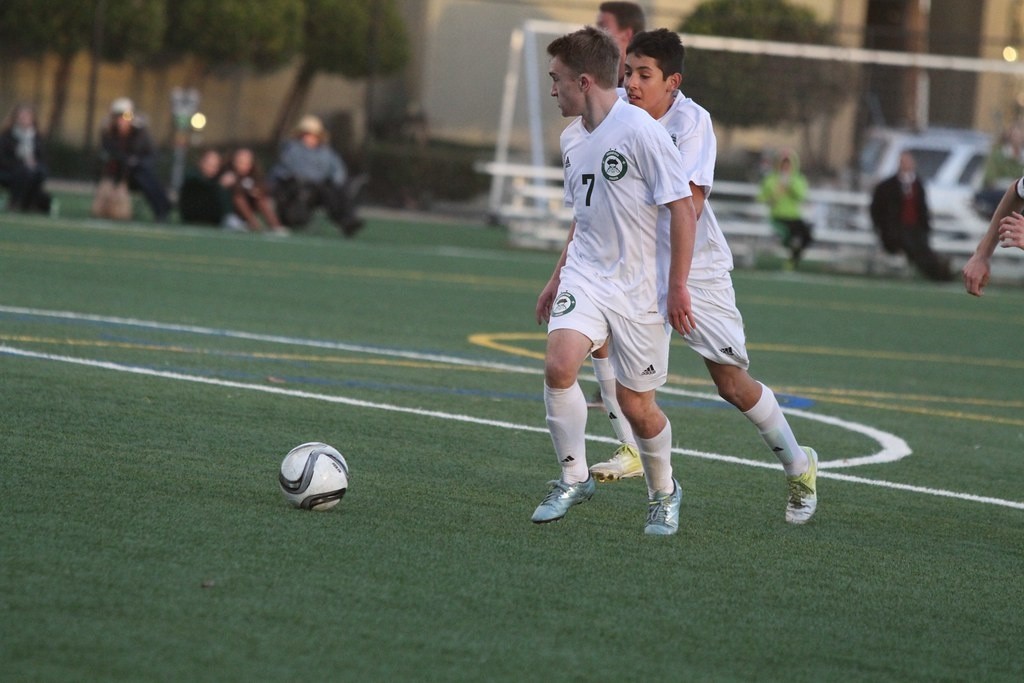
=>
[44,180,178,216]
[477,162,1024,278]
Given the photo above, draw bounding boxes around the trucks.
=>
[816,123,1001,245]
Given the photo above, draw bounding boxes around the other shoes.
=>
[343,216,364,238]
[273,226,291,238]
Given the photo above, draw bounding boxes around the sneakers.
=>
[530,469,596,523]
[589,443,645,482]
[643,477,682,535]
[785,446,818,524]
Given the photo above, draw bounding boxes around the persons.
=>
[531,2,818,535]
[871,127,1024,297]
[99,96,367,237]
[0,105,52,215]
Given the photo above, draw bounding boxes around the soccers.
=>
[278,441,350,512]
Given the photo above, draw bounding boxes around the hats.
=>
[110,96,133,115]
[297,115,322,136]
